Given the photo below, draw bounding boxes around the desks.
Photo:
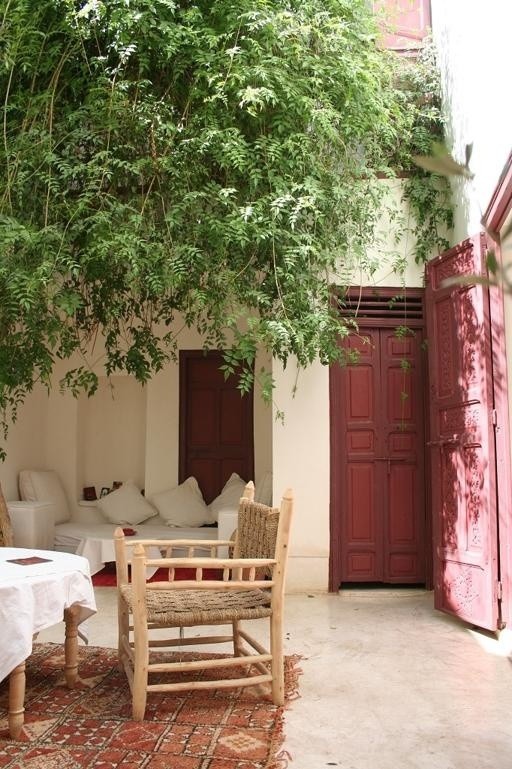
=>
[0,547,97,744]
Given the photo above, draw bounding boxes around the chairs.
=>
[113,480,294,724]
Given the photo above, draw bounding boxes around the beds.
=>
[53,522,219,582]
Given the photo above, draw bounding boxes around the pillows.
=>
[147,476,218,528]
[95,481,159,526]
[19,469,72,525]
[207,472,272,518]
[253,473,272,508]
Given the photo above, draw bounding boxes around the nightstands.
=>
[218,510,237,558]
[6,501,58,550]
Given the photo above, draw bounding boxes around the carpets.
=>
[90,567,223,587]
[0,642,309,769]
